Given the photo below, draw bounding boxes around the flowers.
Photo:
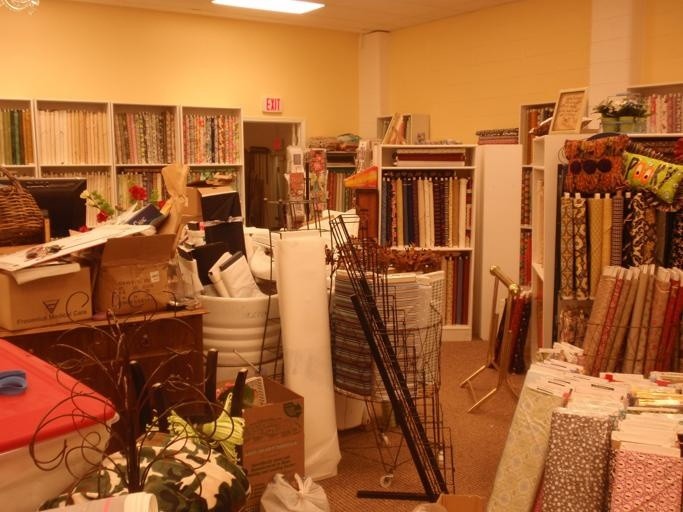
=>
[72,180,167,233]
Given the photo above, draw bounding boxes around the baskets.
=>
[0,166,45,245]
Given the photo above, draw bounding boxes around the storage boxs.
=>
[210,373,312,511]
[67,222,180,314]
[0,238,96,333]
[184,183,205,223]
[0,339,117,512]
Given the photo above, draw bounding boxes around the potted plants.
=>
[620,100,647,132]
[594,98,621,133]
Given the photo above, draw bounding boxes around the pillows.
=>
[38,423,254,512]
[561,132,630,198]
[617,149,683,208]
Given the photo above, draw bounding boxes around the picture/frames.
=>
[544,85,589,135]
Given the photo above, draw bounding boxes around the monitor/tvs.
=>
[0,178,87,244]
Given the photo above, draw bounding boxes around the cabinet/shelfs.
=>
[111,102,182,203]
[474,142,526,342]
[176,103,246,224]
[528,134,592,360]
[1,99,36,182]
[372,110,433,143]
[30,99,115,207]
[624,80,682,139]
[375,144,477,345]
[0,304,213,455]
[319,149,359,212]
